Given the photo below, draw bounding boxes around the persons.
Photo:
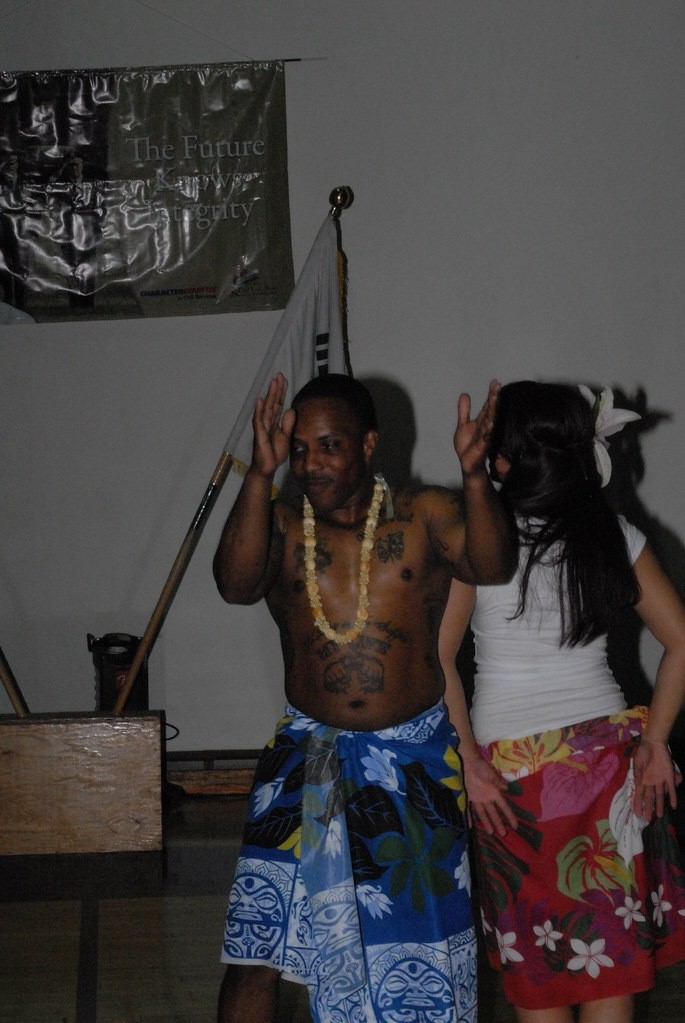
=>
[211,372,513,1023]
[439,375,684,1023]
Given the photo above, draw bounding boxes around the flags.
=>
[220,214,355,537]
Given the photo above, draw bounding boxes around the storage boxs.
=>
[0,712,165,857]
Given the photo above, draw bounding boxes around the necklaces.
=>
[303,472,394,645]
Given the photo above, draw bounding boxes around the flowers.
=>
[575,382,643,495]
